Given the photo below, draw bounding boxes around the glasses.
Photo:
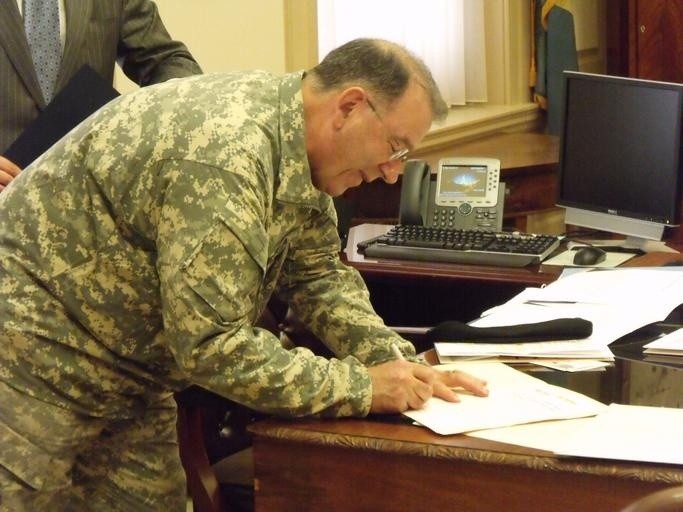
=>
[365,97,409,160]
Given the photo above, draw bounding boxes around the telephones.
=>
[398,157,506,231]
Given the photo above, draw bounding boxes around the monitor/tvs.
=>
[553,70,683,242]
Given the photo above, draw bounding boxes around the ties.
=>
[22,0,61,107]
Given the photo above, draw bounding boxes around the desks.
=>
[249,218,683,510]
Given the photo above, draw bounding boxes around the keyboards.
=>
[362,223,560,268]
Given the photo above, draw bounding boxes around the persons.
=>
[0,37,490,511]
[0,0,204,194]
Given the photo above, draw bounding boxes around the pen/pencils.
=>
[390,343,406,362]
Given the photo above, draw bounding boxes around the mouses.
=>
[573,246,606,265]
